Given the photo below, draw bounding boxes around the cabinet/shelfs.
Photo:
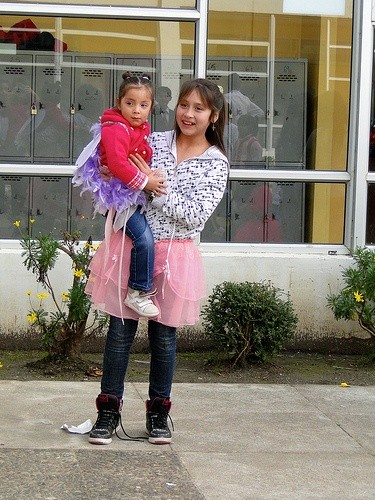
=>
[0,50,308,244]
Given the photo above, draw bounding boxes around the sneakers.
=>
[144,397,173,444]
[87,393,123,445]
[124,286,160,318]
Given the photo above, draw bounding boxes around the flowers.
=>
[325,238,375,342]
[339,382,351,387]
[13,218,111,355]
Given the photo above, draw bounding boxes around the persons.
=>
[1,57,348,246]
[83,79,230,445]
[71,71,168,318]
